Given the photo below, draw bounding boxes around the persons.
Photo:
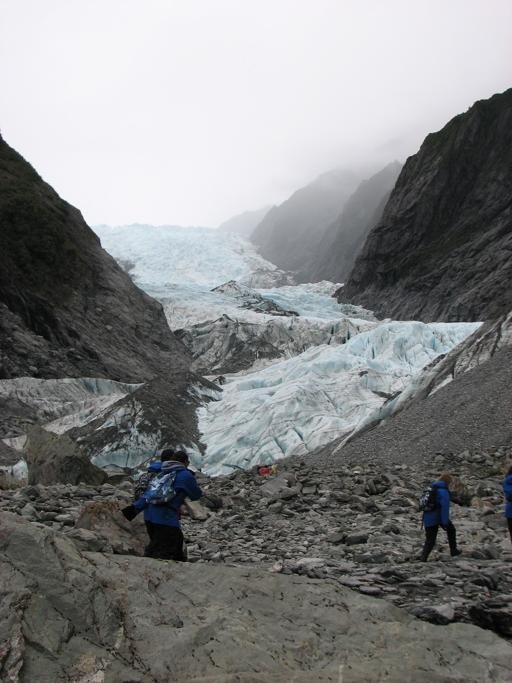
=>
[416,473,463,562]
[502,464,512,545]
[132,448,176,504]
[121,451,204,559]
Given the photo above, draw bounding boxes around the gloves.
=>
[121,506,137,521]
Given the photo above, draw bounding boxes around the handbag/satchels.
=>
[135,472,157,498]
[419,486,449,510]
[145,471,178,504]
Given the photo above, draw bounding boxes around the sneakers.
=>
[419,553,427,561]
[450,548,462,556]
